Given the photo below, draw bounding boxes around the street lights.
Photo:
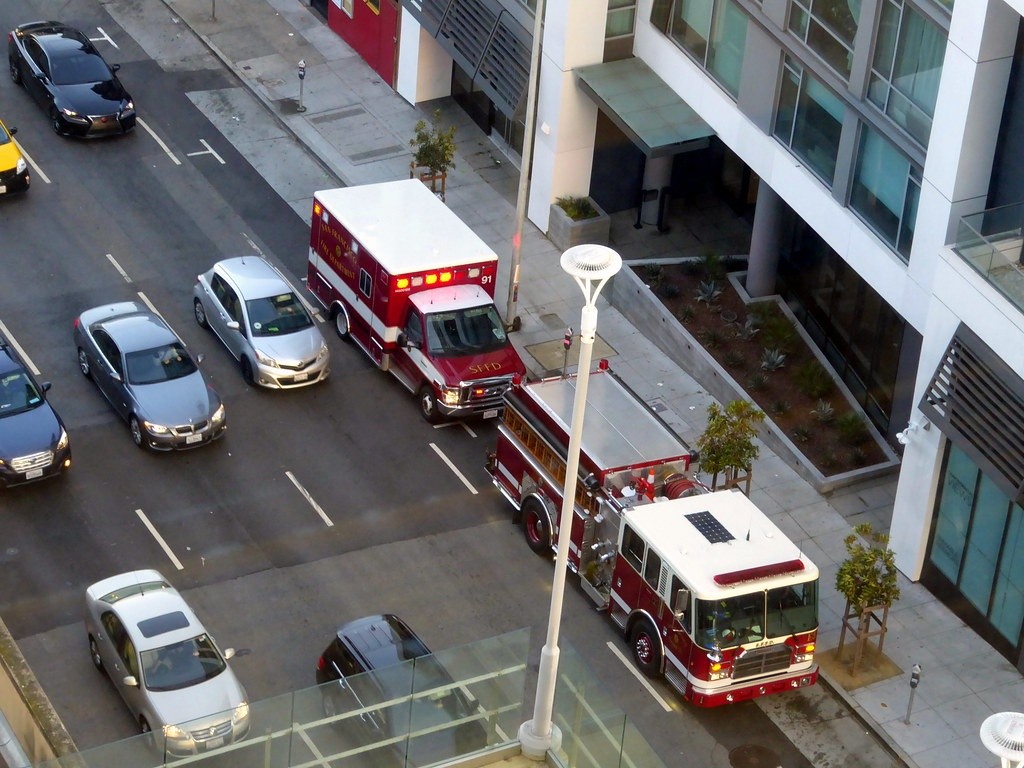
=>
[515,239,625,761]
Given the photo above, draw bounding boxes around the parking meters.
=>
[904,662,922,726]
[296,58,306,112]
[560,326,574,377]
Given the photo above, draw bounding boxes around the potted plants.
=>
[548,194,611,252]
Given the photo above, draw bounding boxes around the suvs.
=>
[314,613,489,768]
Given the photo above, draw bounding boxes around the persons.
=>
[276,302,296,316]
[153,345,181,366]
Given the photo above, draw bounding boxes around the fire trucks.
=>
[301,176,530,425]
[480,357,823,711]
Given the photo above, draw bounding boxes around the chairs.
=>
[109,614,127,651]
[123,637,159,679]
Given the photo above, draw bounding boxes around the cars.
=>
[84,568,254,761]
[72,299,228,453]
[0,119,31,201]
[192,254,332,390]
[8,20,138,139]
[0,333,73,490]
